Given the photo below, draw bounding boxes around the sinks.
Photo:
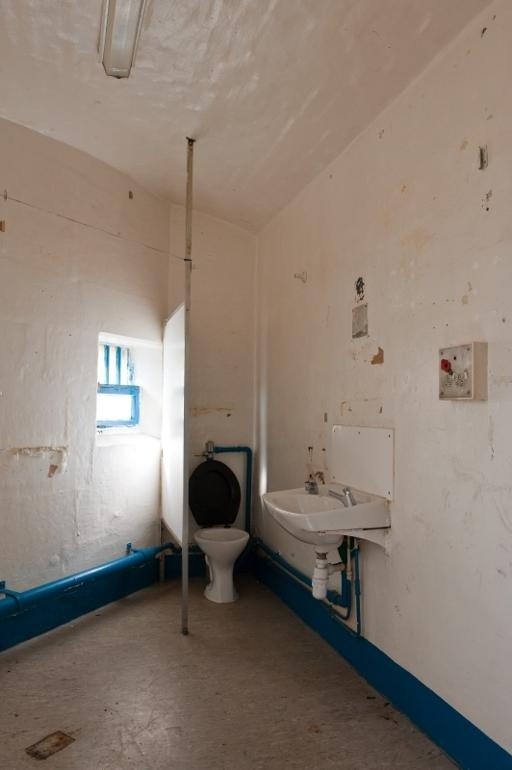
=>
[262,485,390,546]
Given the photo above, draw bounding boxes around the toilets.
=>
[187,459,249,604]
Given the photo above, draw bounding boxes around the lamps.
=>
[95,0,148,81]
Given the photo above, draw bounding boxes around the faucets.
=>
[305,480,319,495]
[328,488,355,507]
